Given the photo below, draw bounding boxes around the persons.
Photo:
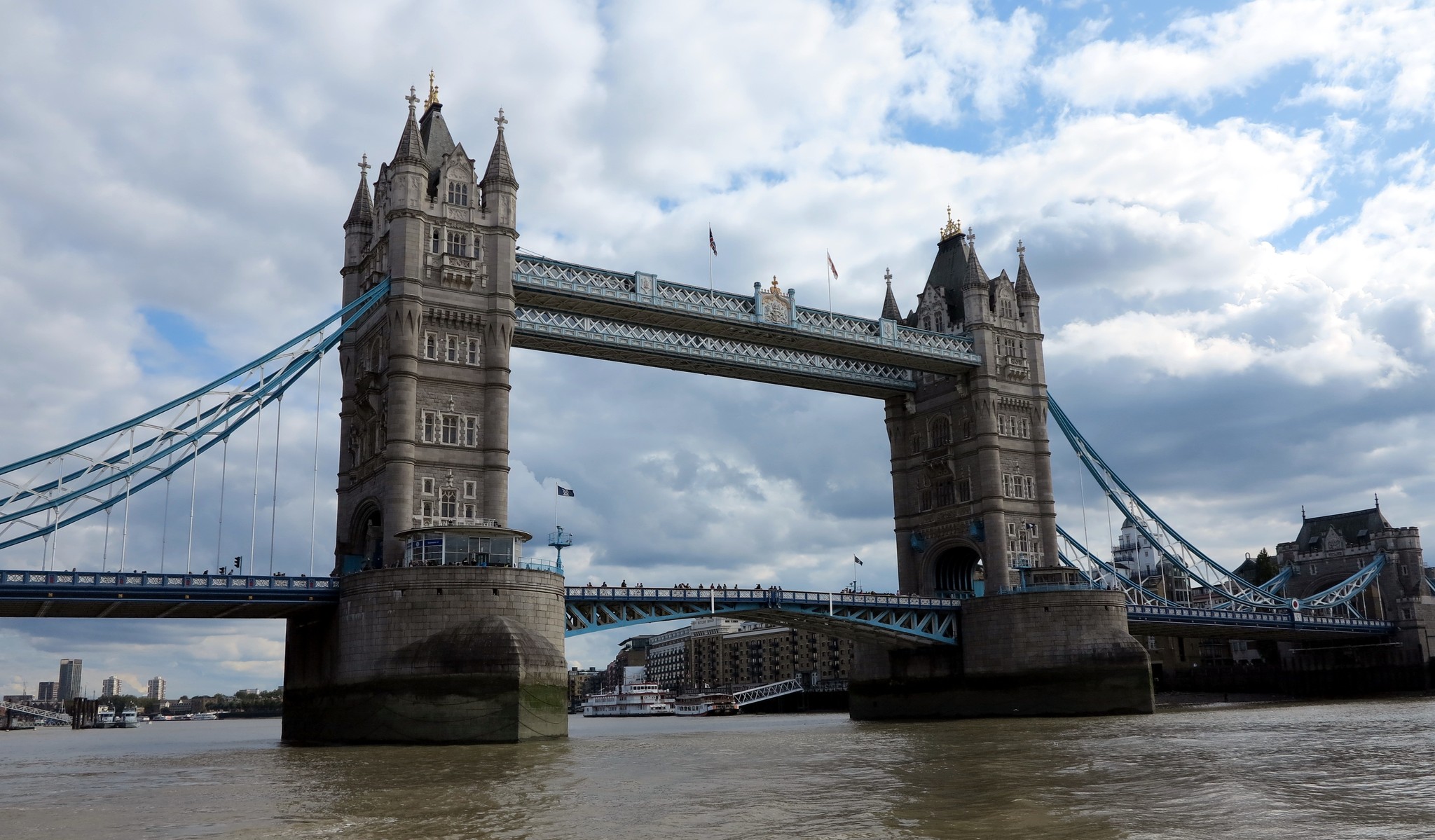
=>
[335,558,552,578]
[1199,605,1211,610]
[970,593,976,598]
[1096,584,1120,591]
[841,589,917,597]
[1267,610,1288,614]
[63,568,306,585]
[951,594,954,599]
[1224,692,1228,702]
[586,580,783,598]
[1302,608,1383,620]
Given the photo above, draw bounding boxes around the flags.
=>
[1025,523,1035,530]
[557,485,574,497]
[705,683,710,688]
[709,226,717,257]
[855,555,863,566]
[695,683,697,689]
[827,251,838,280]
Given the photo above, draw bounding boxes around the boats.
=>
[97,712,118,728]
[118,710,140,728]
[675,693,739,716]
[581,684,676,717]
[137,716,150,721]
[152,715,194,720]
[193,713,217,720]
